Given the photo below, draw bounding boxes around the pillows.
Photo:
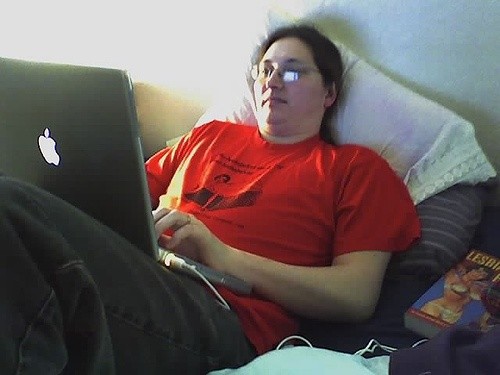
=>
[387,185,483,286]
[194,39,498,206]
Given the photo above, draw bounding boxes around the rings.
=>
[187,214,191,224]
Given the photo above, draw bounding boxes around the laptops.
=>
[1,57,254,298]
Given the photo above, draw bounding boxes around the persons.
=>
[0,26,421,375]
[420,265,492,326]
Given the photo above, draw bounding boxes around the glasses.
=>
[250,62,317,82]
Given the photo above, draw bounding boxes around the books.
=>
[403,248,500,339]
